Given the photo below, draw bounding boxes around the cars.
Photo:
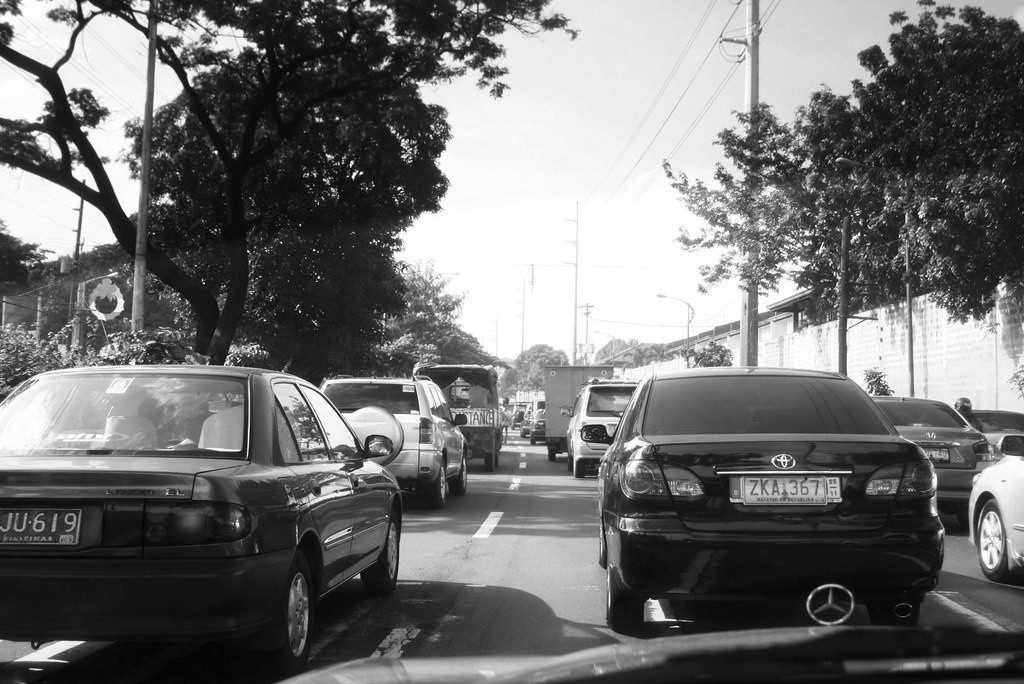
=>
[580,367,944,633]
[520,410,531,438]
[0,364,401,679]
[869,396,1004,529]
[510,411,524,430]
[968,433,1024,582]
[529,409,545,445]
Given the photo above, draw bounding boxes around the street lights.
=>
[657,294,694,349]
[73,271,122,345]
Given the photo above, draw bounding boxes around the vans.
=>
[413,364,503,471]
[532,394,546,416]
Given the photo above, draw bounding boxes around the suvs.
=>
[317,375,468,510]
[561,378,639,478]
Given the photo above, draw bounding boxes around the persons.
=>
[502,406,512,443]
[955,397,983,433]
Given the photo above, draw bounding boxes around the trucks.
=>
[544,366,613,462]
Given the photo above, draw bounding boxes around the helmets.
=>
[955,397,972,411]
